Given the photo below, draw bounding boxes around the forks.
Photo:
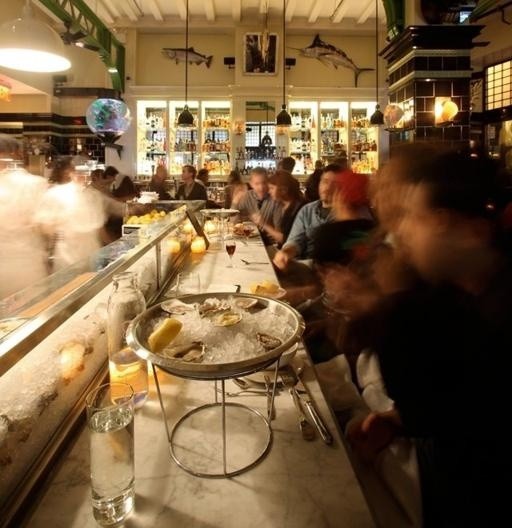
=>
[211,389,277,400]
[265,374,276,423]
[279,369,315,441]
[242,259,269,266]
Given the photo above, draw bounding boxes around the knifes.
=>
[293,377,336,447]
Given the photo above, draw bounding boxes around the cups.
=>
[85,381,135,527]
[176,271,201,295]
[243,222,255,244]
[226,237,236,268]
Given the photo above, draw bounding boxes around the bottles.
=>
[105,271,150,412]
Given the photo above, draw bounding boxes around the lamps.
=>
[175,2,197,128]
[262,105,274,148]
[2,4,73,77]
[85,97,133,143]
[275,1,292,126]
[370,1,385,126]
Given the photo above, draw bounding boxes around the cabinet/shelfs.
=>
[132,97,233,181]
[287,100,377,179]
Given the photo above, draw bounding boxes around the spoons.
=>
[230,379,274,392]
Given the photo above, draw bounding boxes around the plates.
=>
[124,290,304,381]
[268,286,286,299]
[200,210,239,218]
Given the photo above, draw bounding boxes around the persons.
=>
[252,172,307,258]
[315,144,512,528]
[314,172,379,267]
[144,165,173,201]
[272,164,347,276]
[0,137,134,293]
[175,165,208,201]
[229,167,281,244]
